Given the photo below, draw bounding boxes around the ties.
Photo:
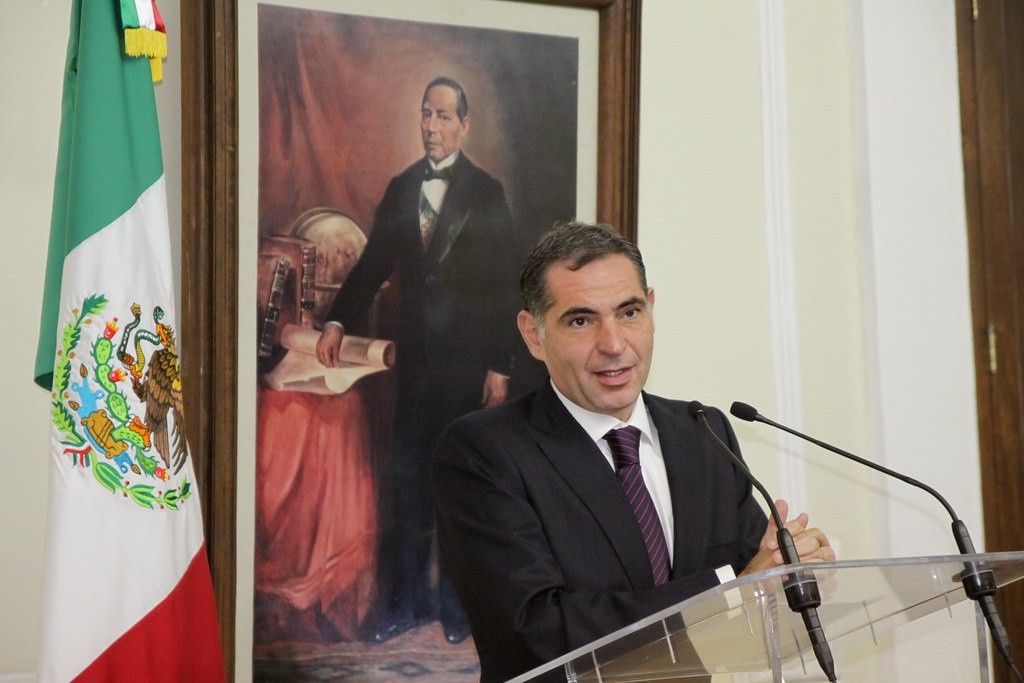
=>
[601,427,671,588]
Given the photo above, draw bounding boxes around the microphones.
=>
[687,401,800,566]
[729,401,977,556]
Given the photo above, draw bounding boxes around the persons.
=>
[432,220,839,683]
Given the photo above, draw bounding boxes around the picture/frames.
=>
[179,0,643,682]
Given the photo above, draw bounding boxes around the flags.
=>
[33,0,222,683]
